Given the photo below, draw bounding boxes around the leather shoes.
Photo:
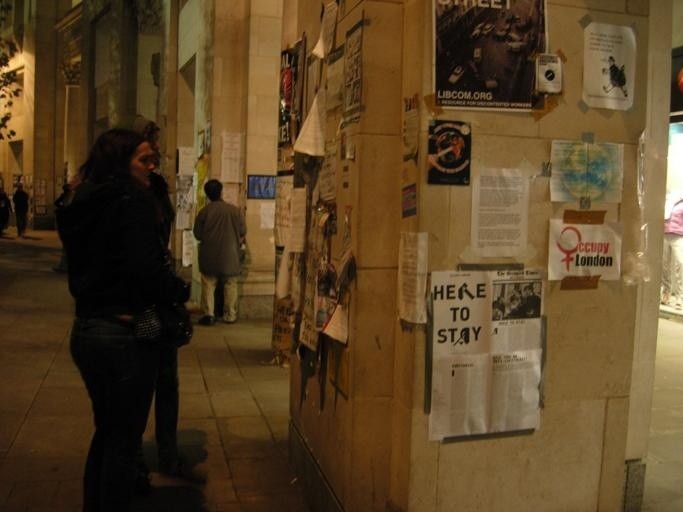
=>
[200,317,215,325]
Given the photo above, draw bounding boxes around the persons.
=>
[193,179,248,323]
[126,111,199,477]
[50,129,209,511]
[439,133,464,162]
[600,54,630,98]
[53,184,74,272]
[13,182,29,241]
[1,187,13,237]
[492,284,541,320]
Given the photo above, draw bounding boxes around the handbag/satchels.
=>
[130,302,194,359]
